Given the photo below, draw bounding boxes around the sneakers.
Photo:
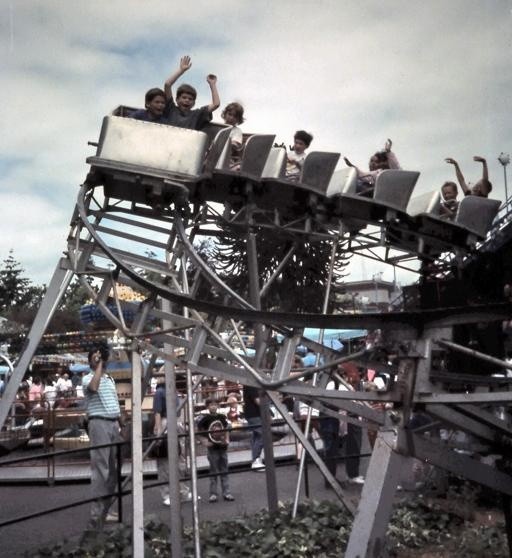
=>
[251,457,266,472]
[208,493,218,503]
[324,479,346,490]
[161,495,171,507]
[223,493,235,501]
[346,476,365,486]
[180,492,202,503]
[103,511,119,523]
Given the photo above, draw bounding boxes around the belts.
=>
[88,414,119,423]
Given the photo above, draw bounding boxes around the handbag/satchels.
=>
[149,432,182,460]
